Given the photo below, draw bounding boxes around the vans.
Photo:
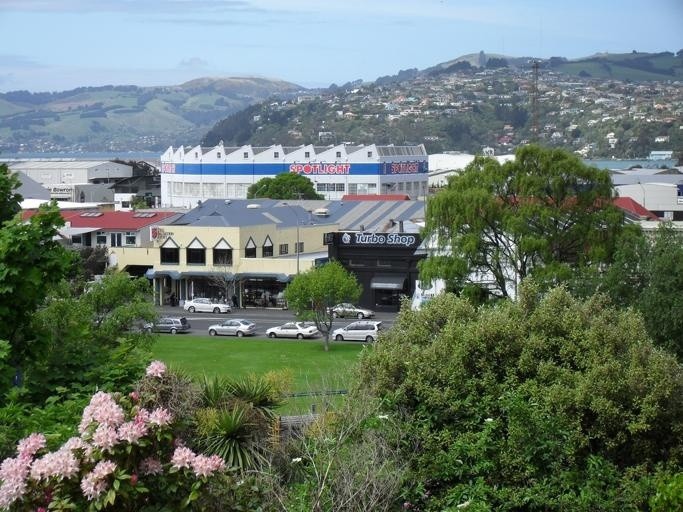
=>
[331,320,383,342]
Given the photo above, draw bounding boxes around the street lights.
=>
[280,202,300,277]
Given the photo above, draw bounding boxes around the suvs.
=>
[144,317,191,334]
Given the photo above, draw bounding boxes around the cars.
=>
[266,320,318,341]
[244,289,271,300]
[207,319,257,337]
[326,302,375,318]
[183,297,231,313]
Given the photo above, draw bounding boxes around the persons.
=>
[231,293,239,308]
[169,291,176,306]
